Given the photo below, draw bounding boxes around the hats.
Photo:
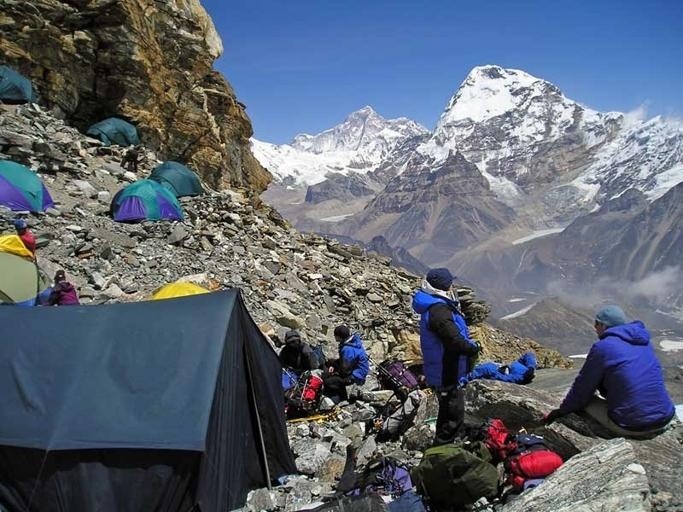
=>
[426,267,458,292]
[592,303,626,328]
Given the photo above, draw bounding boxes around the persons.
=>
[14,219,35,254]
[275,330,319,374]
[322,326,368,393]
[49,270,79,305]
[542,307,673,440]
[413,268,481,443]
[121,149,139,172]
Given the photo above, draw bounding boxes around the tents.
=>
[147,280,212,301]
[0,288,296,512]
[86,117,142,147]
[0,65,37,103]
[1,160,55,212]
[0,249,52,305]
[109,179,184,222]
[149,162,205,195]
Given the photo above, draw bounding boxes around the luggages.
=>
[366,354,420,395]
[291,369,323,413]
